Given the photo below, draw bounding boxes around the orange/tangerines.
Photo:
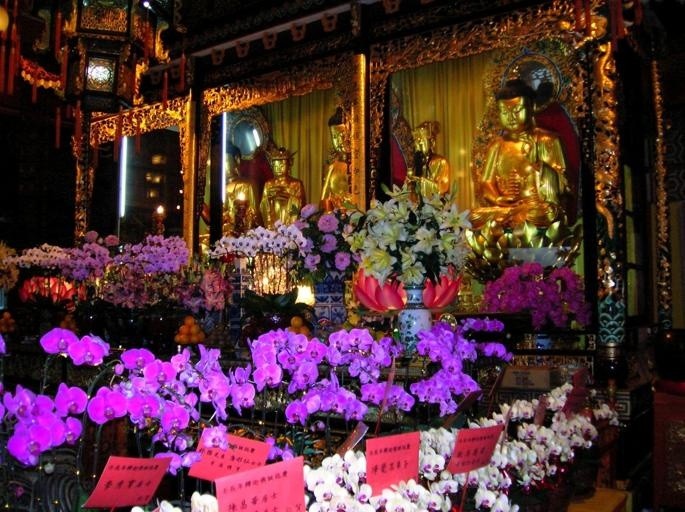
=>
[0,312,16,333]
[61,315,76,334]
[174,316,205,344]
[287,316,312,341]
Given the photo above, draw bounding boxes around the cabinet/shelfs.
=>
[649,379,685,510]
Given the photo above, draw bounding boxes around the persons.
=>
[258,145,307,231]
[466,79,570,264]
[223,139,258,236]
[318,105,351,213]
[400,120,452,202]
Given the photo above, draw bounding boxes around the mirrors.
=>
[220,106,273,165]
